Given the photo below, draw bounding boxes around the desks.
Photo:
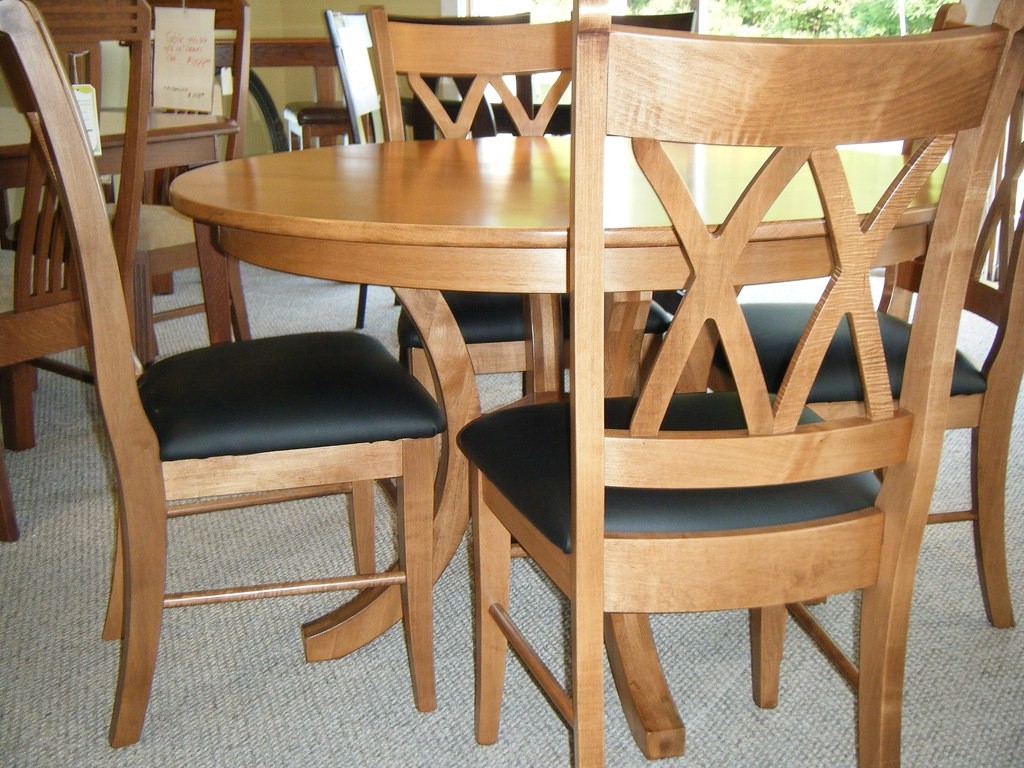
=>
[115,37,342,148]
[0,107,242,451]
[397,77,572,136]
[165,137,956,759]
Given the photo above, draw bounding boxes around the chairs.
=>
[0,0,1024,768]
